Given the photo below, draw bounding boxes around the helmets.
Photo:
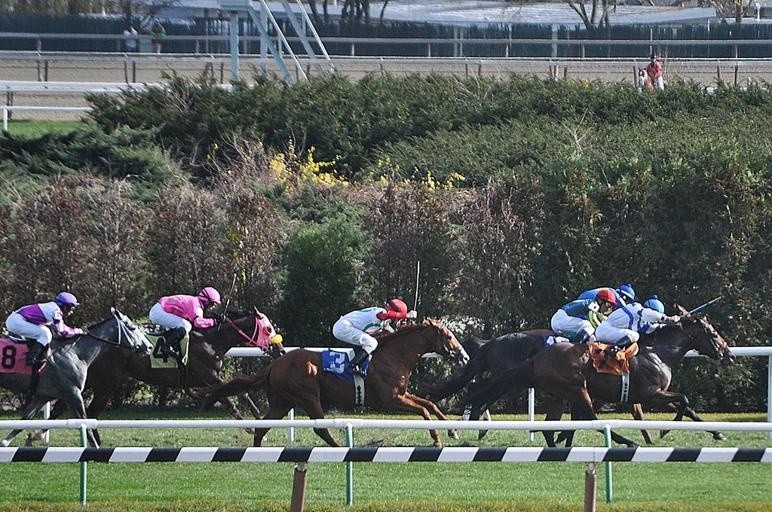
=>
[56,292,78,307]
[644,295,665,313]
[598,289,617,305]
[618,283,635,300]
[385,298,407,314]
[199,286,221,303]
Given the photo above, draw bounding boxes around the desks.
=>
[0,81,255,120]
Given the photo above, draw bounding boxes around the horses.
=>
[636,68,669,94]
[196,313,470,448]
[429,303,737,448]
[0,305,285,447]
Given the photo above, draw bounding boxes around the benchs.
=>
[0,106,91,131]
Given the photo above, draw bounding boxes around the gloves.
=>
[216,315,224,322]
[407,308,418,319]
[670,315,681,324]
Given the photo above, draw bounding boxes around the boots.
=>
[160,328,185,357]
[609,337,631,360]
[27,343,45,367]
[348,349,369,375]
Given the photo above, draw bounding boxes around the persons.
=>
[333,298,417,373]
[123,25,138,52]
[550,290,617,344]
[6,291,88,365]
[595,298,682,362]
[645,55,662,89]
[576,284,635,311]
[148,18,166,52]
[148,285,223,356]
